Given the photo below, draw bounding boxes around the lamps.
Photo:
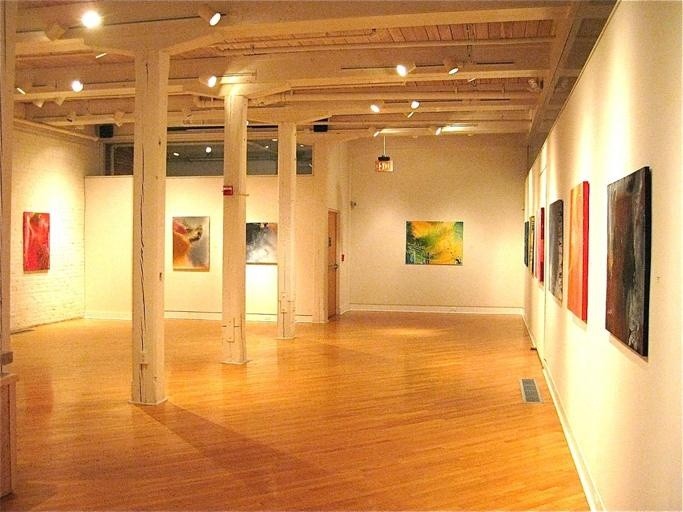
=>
[367,55,476,138]
[112,108,126,127]
[33,99,44,108]
[375,136,393,173]
[54,96,66,106]
[197,3,227,26]
[180,104,193,119]
[198,72,217,88]
[67,112,79,122]
